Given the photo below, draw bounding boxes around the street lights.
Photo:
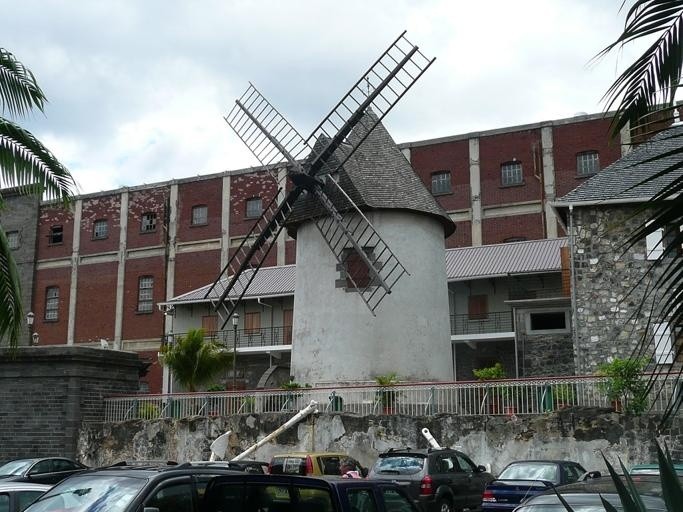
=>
[232,311,241,390]
[25,310,36,346]
[167,328,175,394]
[32,331,40,346]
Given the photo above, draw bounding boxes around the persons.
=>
[339,456,361,479]
